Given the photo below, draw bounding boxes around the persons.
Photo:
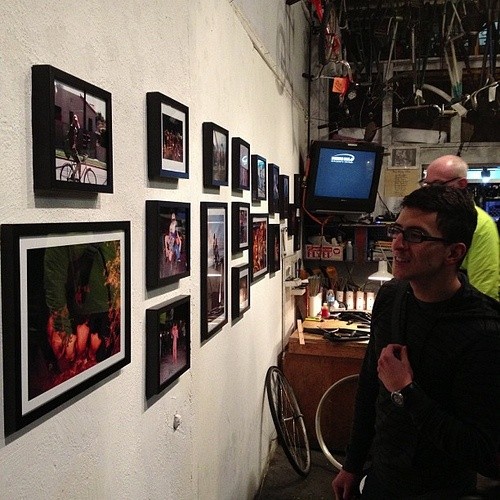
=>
[421,155,500,300]
[168,212,184,269]
[332,184,499,500]
[67,114,83,181]
[170,323,178,364]
[212,232,220,270]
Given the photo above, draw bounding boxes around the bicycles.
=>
[59,152,98,184]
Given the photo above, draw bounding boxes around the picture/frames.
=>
[231,201,250,255]
[231,136,251,191]
[1,220,132,439]
[147,92,190,181]
[269,223,284,274]
[202,121,229,188]
[281,174,291,219]
[145,199,191,293]
[268,163,281,213]
[146,295,191,400]
[32,64,114,194]
[250,213,269,283]
[200,201,228,342]
[388,145,422,170]
[231,263,250,319]
[251,155,268,201]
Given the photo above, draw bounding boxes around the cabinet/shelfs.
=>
[296,220,399,322]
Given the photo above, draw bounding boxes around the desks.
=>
[280,304,373,458]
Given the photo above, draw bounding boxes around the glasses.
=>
[389,224,445,243]
[419,177,461,189]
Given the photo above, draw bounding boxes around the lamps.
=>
[367,260,395,286]
[480,167,493,186]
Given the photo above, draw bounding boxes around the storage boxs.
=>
[304,244,321,260]
[320,245,344,262]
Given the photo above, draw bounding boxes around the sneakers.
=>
[78,177,81,182]
[70,173,76,180]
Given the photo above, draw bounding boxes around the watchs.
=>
[391,382,417,407]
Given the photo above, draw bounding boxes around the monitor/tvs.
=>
[304,140,384,213]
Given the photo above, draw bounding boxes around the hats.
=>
[171,213,176,220]
[73,115,78,121]
